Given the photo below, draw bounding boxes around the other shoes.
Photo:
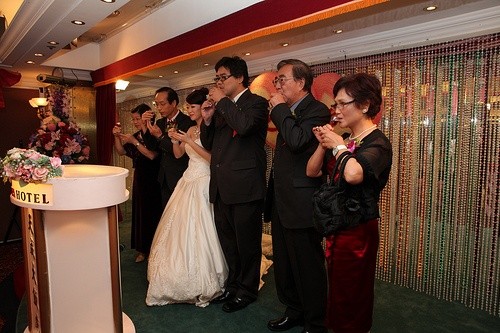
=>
[136,256,146,263]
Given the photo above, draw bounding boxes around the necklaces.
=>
[194,126,198,138]
[349,125,377,140]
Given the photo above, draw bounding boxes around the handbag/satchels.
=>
[311,152,381,236]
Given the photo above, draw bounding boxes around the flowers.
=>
[345,138,364,153]
[166,119,179,130]
[0,147,65,185]
[27,114,90,165]
[232,129,237,138]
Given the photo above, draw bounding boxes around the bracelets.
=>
[136,142,139,147]
[171,140,178,144]
[157,134,164,142]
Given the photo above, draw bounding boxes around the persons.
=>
[145,87,228,307]
[142,87,197,260]
[113,104,164,261]
[264,58,332,333]
[200,56,270,313]
[306,72,393,333]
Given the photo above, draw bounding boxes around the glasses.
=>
[214,73,235,82]
[272,77,300,86]
[153,100,173,107]
[332,98,360,110]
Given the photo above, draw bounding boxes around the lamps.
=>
[29,97,50,121]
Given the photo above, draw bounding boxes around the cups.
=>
[169,119,178,128]
[330,116,338,127]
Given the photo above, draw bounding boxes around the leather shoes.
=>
[300,324,327,333]
[209,289,233,303]
[267,314,307,331]
[222,293,258,312]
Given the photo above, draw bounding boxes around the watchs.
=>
[333,145,347,156]
